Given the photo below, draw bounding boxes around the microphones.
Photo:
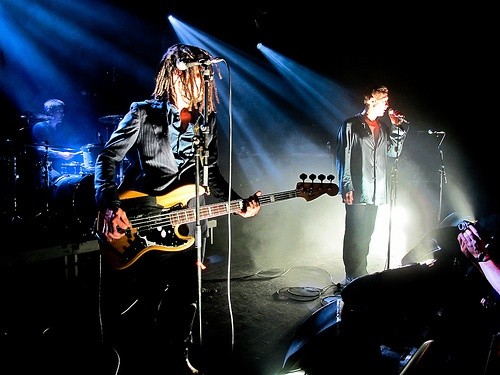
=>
[428,130,446,135]
[388,108,409,124]
[175,57,224,70]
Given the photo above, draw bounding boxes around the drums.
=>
[82,143,108,168]
[61,161,82,176]
[49,173,97,228]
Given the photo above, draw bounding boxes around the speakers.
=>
[283,298,342,375]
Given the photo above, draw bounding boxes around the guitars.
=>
[97,172,340,266]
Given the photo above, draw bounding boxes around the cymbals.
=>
[26,143,59,147]
[98,113,125,124]
[48,147,80,156]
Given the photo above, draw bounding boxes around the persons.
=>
[448,222,500,305]
[32,99,74,181]
[337,85,404,285]
[93,43,262,375]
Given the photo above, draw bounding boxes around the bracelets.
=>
[474,252,490,262]
